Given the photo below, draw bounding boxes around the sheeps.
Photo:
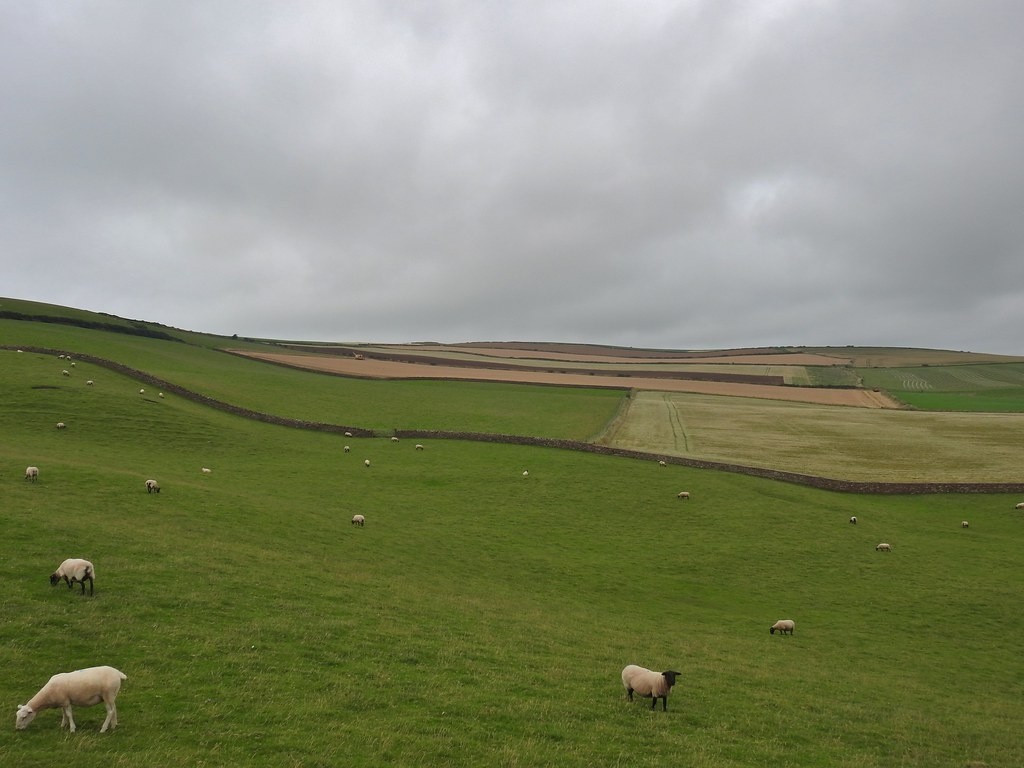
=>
[87,381,93,385]
[351,514,365,528]
[202,468,212,473]
[62,369,69,375]
[1015,503,1024,509]
[56,422,67,429]
[876,543,891,552]
[343,432,423,466]
[523,471,528,477]
[678,492,690,500]
[159,392,164,398]
[58,354,76,367]
[145,479,161,494]
[18,349,23,352]
[961,521,968,527]
[769,619,795,636]
[850,517,857,524]
[140,389,145,393]
[659,460,666,467]
[24,467,39,483]
[50,559,94,597]
[16,665,128,734]
[621,664,681,711]
